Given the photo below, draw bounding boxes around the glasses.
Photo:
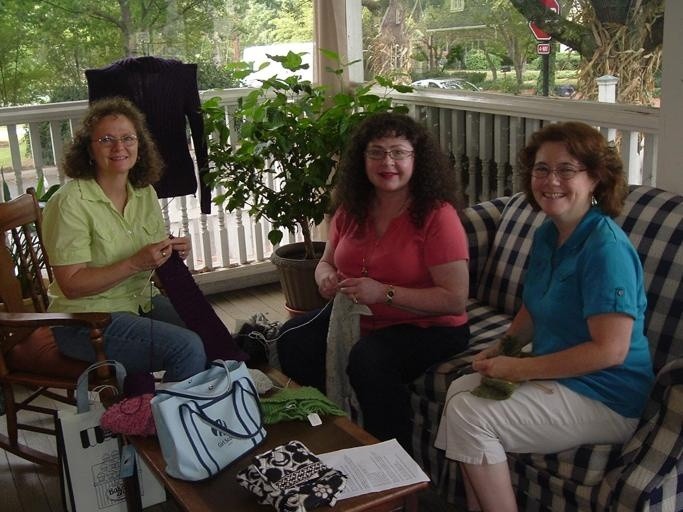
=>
[529,164,588,180]
[362,149,413,160]
[88,134,139,146]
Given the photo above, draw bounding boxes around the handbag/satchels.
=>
[235,312,283,369]
[236,440,347,512]
[151,359,267,483]
[55,359,167,511]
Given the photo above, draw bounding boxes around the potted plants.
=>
[186,45,413,312]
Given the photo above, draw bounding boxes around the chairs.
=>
[0,187,124,473]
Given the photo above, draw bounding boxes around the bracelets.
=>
[384,284,395,306]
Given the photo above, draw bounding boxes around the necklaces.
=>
[357,196,409,278]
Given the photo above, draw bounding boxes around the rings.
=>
[160,250,166,257]
[181,251,184,256]
[351,292,360,305]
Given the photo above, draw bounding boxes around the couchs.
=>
[391,184,681,512]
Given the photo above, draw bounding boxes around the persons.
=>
[39,97,209,385]
[433,119,657,511]
[273,110,472,457]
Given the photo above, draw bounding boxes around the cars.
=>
[552,85,576,98]
[411,77,478,93]
